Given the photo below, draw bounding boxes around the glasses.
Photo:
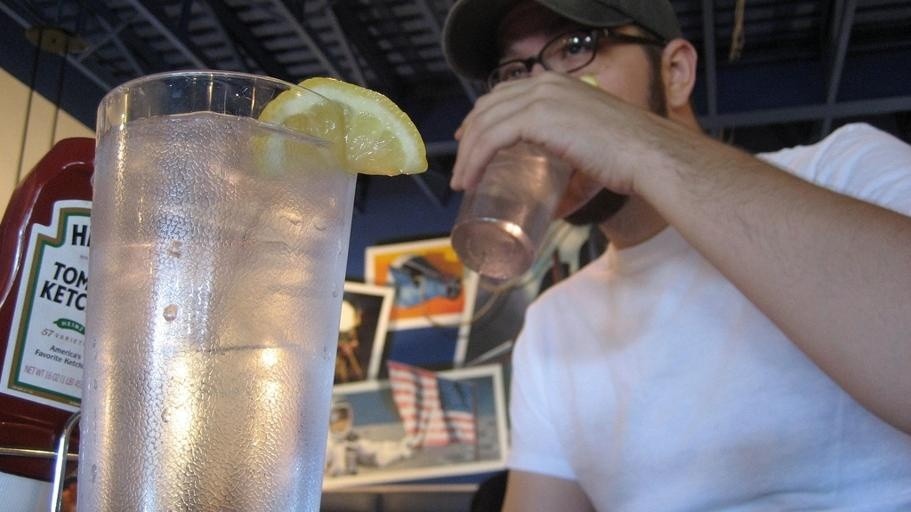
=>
[483,28,668,99]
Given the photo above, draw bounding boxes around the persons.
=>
[442,0,911,512]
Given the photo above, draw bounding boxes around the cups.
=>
[451,134,578,285]
[71,69,361,511]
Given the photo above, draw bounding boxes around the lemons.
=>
[251,76,428,175]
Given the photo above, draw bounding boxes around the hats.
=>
[440,1,686,79]
